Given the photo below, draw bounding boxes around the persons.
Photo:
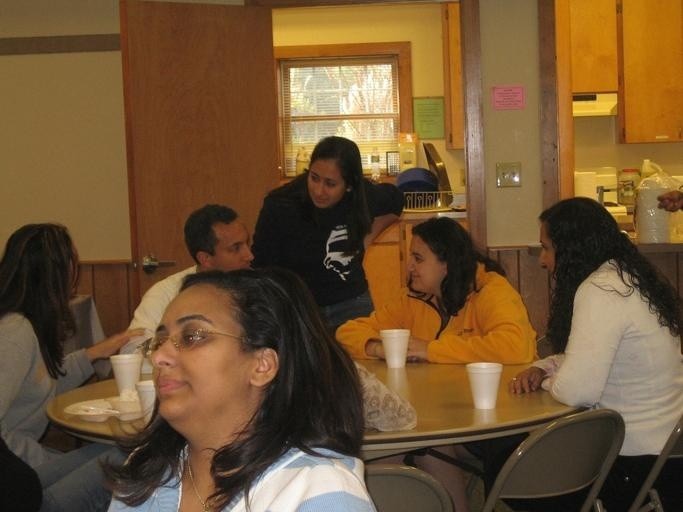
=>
[333,215,543,510]
[0,436,126,512]
[0,221,146,465]
[249,134,406,330]
[114,202,256,374]
[95,265,376,512]
[505,195,683,512]
[255,267,418,440]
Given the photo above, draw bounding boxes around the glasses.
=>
[145,327,247,350]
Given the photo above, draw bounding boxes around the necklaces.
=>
[180,442,234,512]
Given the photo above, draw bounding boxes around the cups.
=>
[381,329,411,368]
[111,354,144,393]
[467,363,503,410]
[137,380,156,427]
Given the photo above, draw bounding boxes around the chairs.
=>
[629,413,682,508]
[481,406,626,508]
[362,462,454,508]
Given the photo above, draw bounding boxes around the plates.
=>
[65,397,118,423]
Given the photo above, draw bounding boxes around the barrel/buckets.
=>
[619,169,641,206]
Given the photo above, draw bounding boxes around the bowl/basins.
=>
[423,141,453,206]
[393,167,438,207]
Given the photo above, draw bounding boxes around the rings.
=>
[511,374,519,381]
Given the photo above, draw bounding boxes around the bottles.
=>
[370,146,380,183]
